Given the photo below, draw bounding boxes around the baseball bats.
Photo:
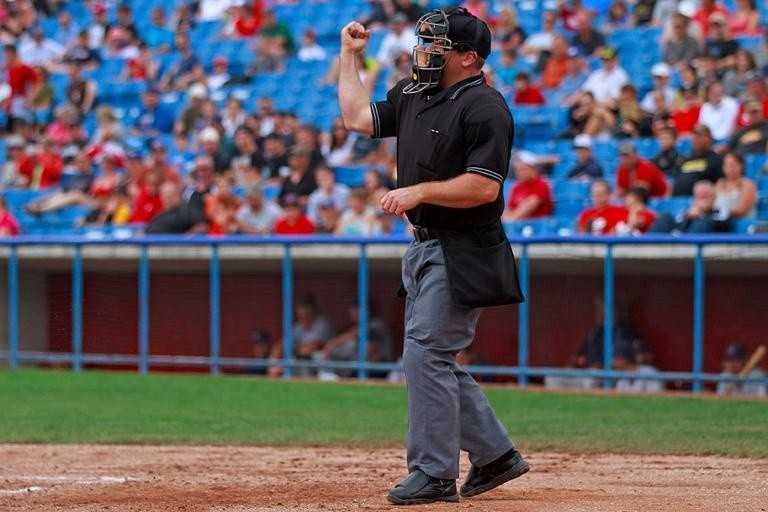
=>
[724,345,766,396]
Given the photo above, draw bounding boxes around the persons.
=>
[0,1,768,235]
[249,292,495,385]
[338,6,530,505]
[543,299,767,395]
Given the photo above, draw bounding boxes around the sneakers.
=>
[460,450,529,497]
[387,469,459,505]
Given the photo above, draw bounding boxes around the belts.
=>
[412,226,439,243]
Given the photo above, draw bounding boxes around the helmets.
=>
[401,8,491,94]
